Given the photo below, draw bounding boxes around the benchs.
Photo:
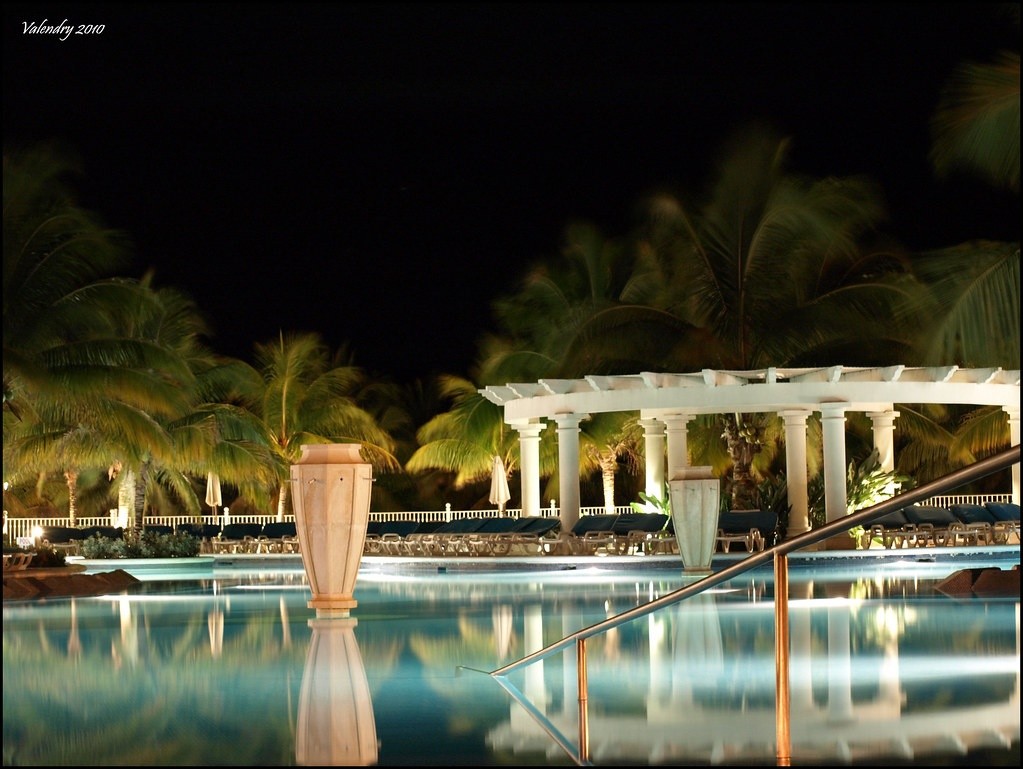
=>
[851,500,1021,549]
[4,508,789,571]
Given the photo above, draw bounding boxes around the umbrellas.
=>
[204,470,222,516]
[488,455,511,518]
